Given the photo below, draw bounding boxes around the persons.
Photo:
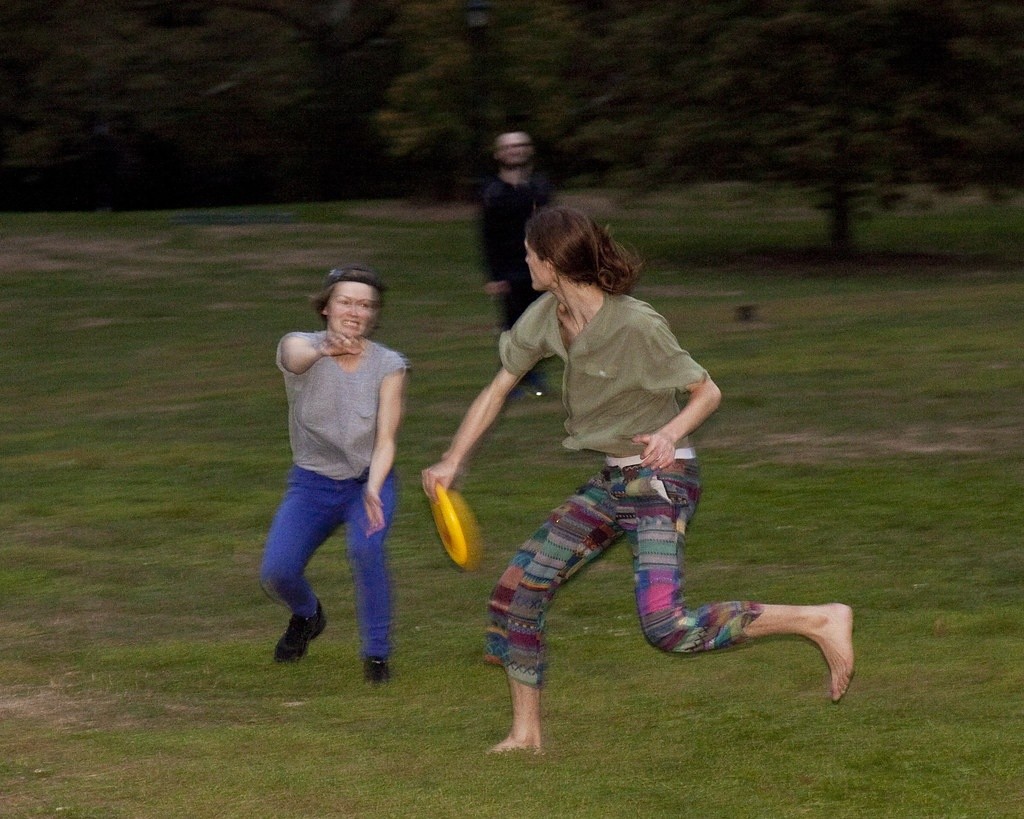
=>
[262,264,407,681]
[477,131,549,398]
[424,198,853,755]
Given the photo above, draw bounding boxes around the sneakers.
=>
[271,600,325,660]
[363,654,388,686]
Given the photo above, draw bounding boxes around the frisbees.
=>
[426,483,471,570]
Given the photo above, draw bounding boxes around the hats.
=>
[318,262,384,293]
